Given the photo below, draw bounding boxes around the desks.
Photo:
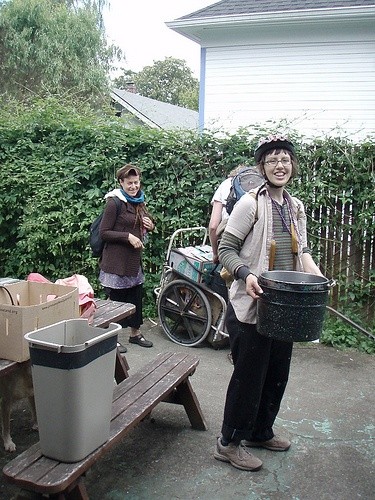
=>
[0,297,136,385]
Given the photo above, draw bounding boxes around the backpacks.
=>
[89,197,121,258]
[224,168,265,214]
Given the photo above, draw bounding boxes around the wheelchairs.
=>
[152,227,230,351]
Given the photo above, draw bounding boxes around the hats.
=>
[116,164,141,183]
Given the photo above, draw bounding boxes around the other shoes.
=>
[116,342,127,352]
[129,333,153,347]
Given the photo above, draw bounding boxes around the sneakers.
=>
[241,434,291,451]
[214,435,262,471]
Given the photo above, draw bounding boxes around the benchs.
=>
[1,352,210,500]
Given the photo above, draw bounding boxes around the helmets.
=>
[254,132,296,164]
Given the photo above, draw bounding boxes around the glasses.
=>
[264,156,293,167]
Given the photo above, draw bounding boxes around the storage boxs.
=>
[0,281,80,363]
[170,245,217,283]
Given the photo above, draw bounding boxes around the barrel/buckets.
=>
[256,270,333,342]
[207,272,228,304]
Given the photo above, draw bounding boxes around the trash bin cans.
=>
[24,318,123,463]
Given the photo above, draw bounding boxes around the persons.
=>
[212,136,329,472]
[208,166,266,265]
[98,164,156,348]
